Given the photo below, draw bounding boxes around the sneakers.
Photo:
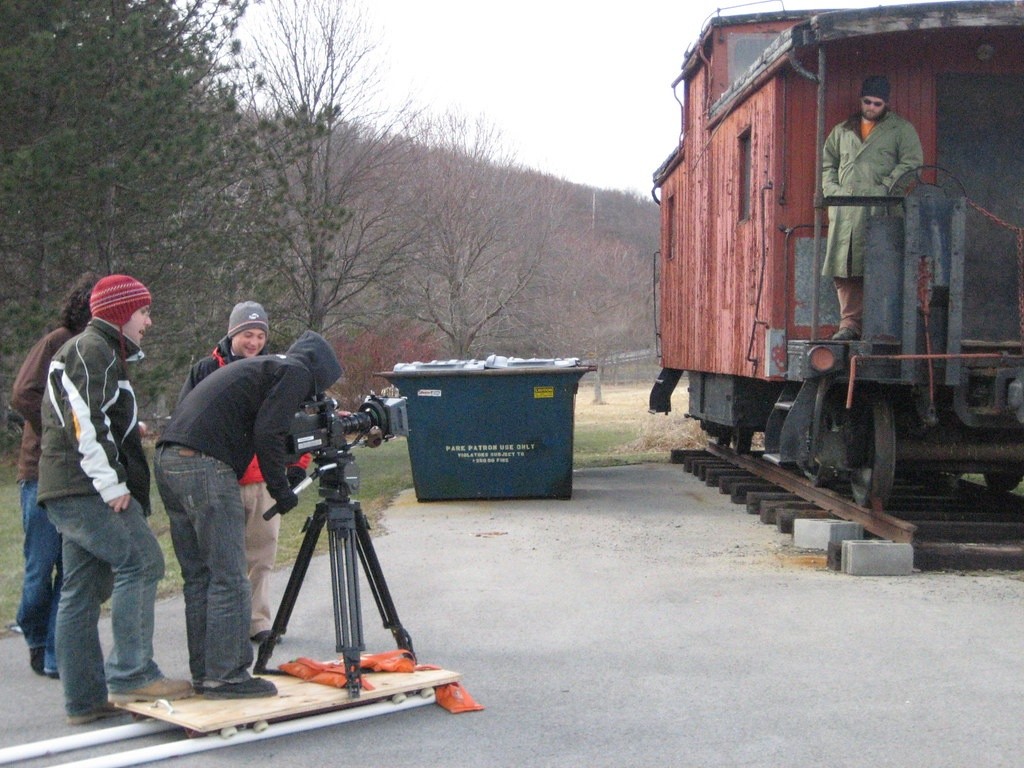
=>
[67,701,127,723]
[108,674,194,704]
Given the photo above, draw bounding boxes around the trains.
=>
[648,0,1024,509]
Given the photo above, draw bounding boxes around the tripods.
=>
[252,448,417,700]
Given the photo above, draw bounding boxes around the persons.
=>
[176,301,311,644]
[155,330,350,696]
[33,275,191,726]
[822,76,923,340]
[11,271,100,677]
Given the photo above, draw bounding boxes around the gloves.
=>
[287,466,306,489]
[276,490,298,514]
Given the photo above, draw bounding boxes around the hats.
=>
[860,75,890,102]
[90,275,152,384]
[228,301,269,340]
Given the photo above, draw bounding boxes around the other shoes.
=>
[203,678,278,700]
[250,630,283,643]
[833,328,856,340]
[30,645,45,676]
[194,684,204,694]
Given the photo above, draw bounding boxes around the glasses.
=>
[859,99,884,107]
